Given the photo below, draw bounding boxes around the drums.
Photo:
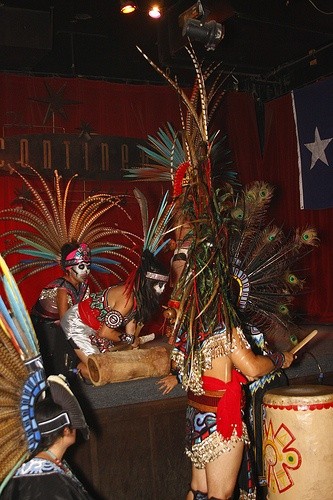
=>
[260,383,332,500]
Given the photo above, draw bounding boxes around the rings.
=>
[294,355,298,360]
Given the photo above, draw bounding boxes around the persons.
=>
[155,358,182,395]
[61,250,170,380]
[0,373,91,500]
[181,266,294,500]
[30,241,91,389]
